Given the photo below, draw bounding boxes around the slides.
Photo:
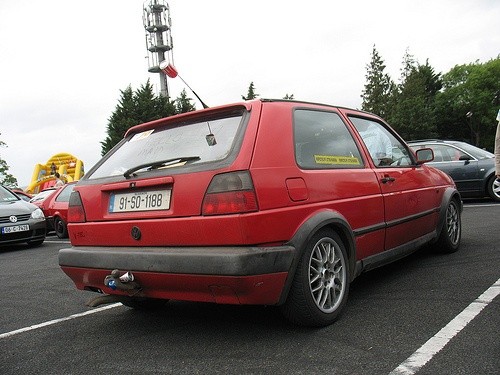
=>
[41,179,58,191]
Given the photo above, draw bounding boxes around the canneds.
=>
[158,59,178,79]
[108,280,117,289]
[120,271,135,284]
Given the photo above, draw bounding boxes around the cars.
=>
[407,140,500,202]
[58,99,463,325]
[0,186,47,249]
[42,181,78,238]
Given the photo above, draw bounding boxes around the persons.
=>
[54,172,64,187]
[356,118,392,166]
[494,108,500,180]
